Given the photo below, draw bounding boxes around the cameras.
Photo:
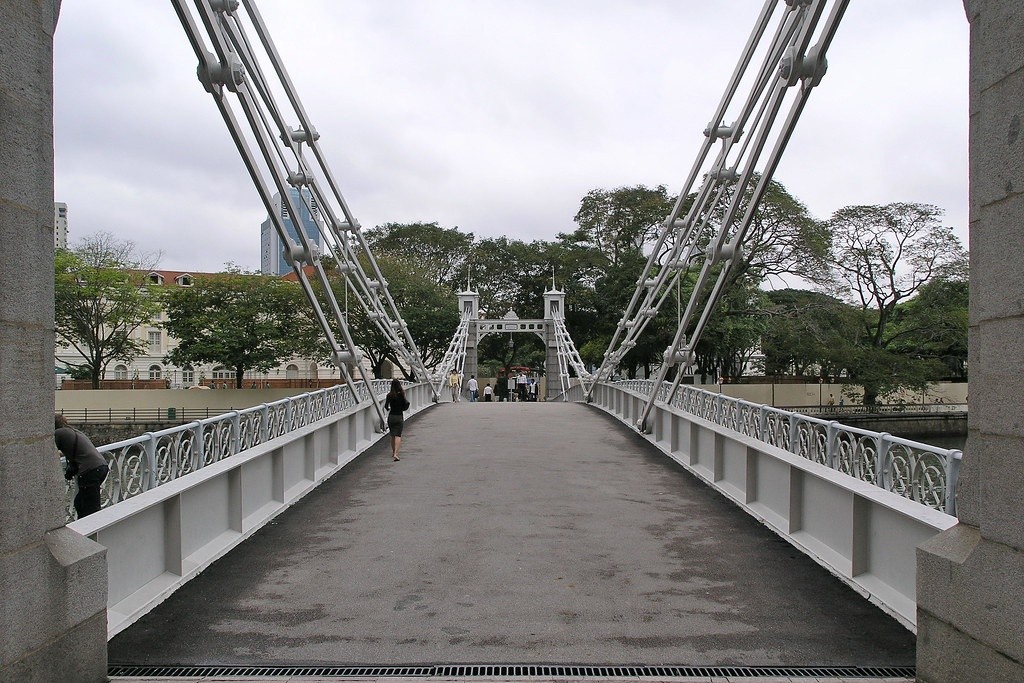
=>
[65,463,78,480]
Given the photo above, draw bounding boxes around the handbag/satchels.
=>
[475,389,480,398]
[402,396,410,411]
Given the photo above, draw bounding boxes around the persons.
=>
[223,382,227,389]
[251,382,256,389]
[517,371,527,402]
[483,383,493,402]
[449,369,461,403]
[55,413,109,518]
[528,378,538,402]
[210,380,215,389]
[467,374,479,402]
[266,382,270,388]
[385,379,406,461]
[309,379,312,388]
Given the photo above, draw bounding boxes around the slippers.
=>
[394,457,400,462]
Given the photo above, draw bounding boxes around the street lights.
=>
[819,377,823,414]
[719,376,723,392]
